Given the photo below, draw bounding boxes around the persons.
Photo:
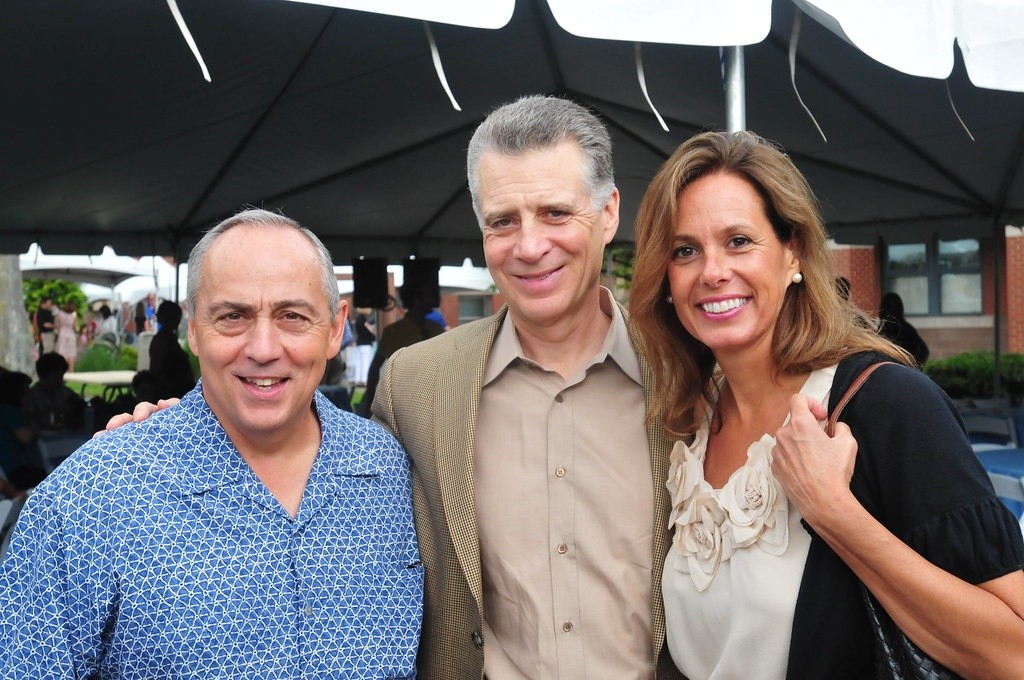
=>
[835,277,851,302]
[91,96,683,680]
[316,353,353,411]
[0,209,425,679]
[367,285,447,411]
[629,132,1024,680]
[878,291,929,367]
[355,315,376,347]
[34,296,119,373]
[132,300,197,403]
[0,351,87,563]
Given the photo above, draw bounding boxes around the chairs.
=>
[36,437,85,475]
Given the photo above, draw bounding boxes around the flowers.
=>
[667,433,789,592]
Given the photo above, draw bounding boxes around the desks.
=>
[972,449,1024,522]
[64,368,143,402]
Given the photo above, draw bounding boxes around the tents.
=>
[18,244,158,313]
[0,0,1023,245]
